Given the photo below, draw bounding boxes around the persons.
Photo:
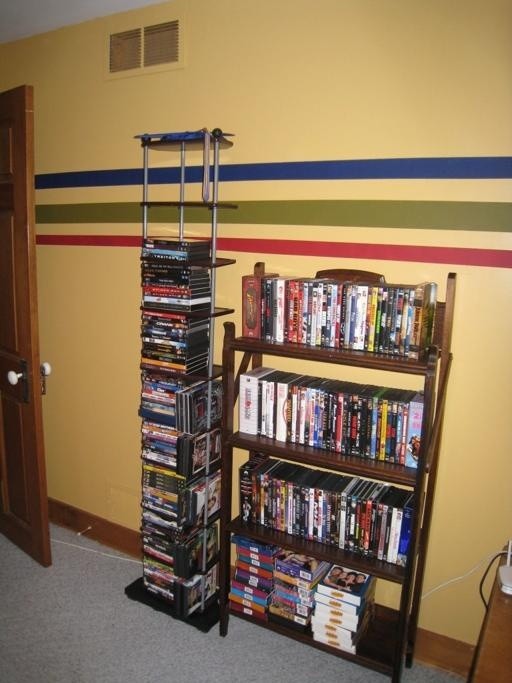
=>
[324,567,367,594]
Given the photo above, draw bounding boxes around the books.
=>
[228,533,375,655]
[136,377,225,435]
[239,458,417,567]
[140,522,219,578]
[140,318,210,373]
[142,570,217,616]
[239,365,423,471]
[241,275,437,364]
[139,427,220,479]
[139,236,212,319]
[141,469,221,528]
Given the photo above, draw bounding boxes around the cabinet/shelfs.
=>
[122,126,237,636]
[215,260,459,683]
